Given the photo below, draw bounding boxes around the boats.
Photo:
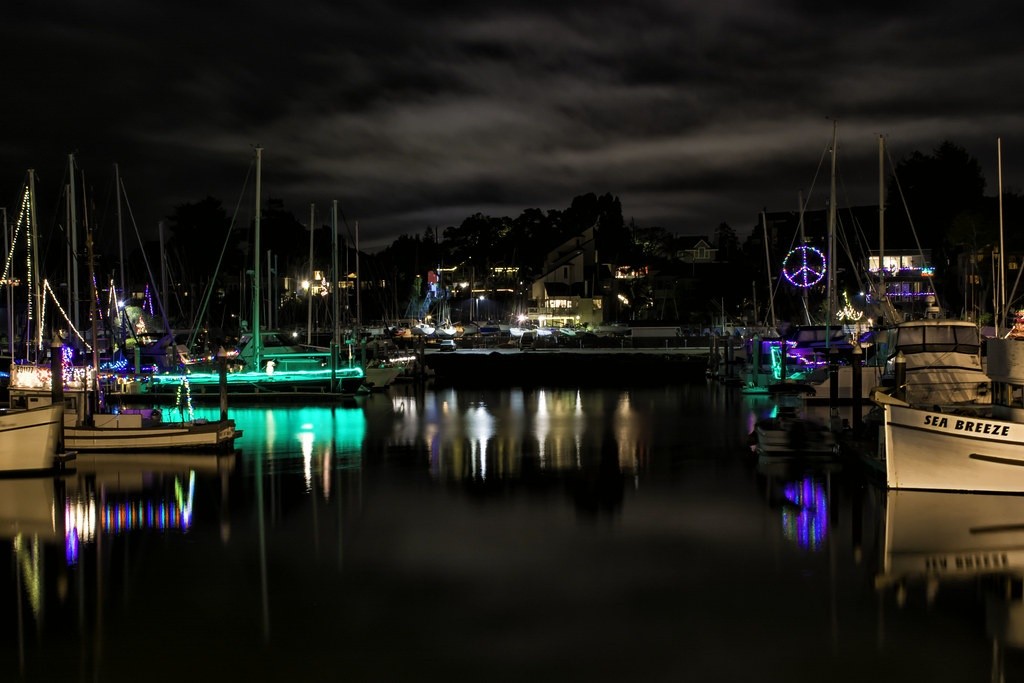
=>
[0,229,243,452]
[7,146,409,405]
[302,115,1023,493]
[826,495,1024,683]
[0,403,64,476]
